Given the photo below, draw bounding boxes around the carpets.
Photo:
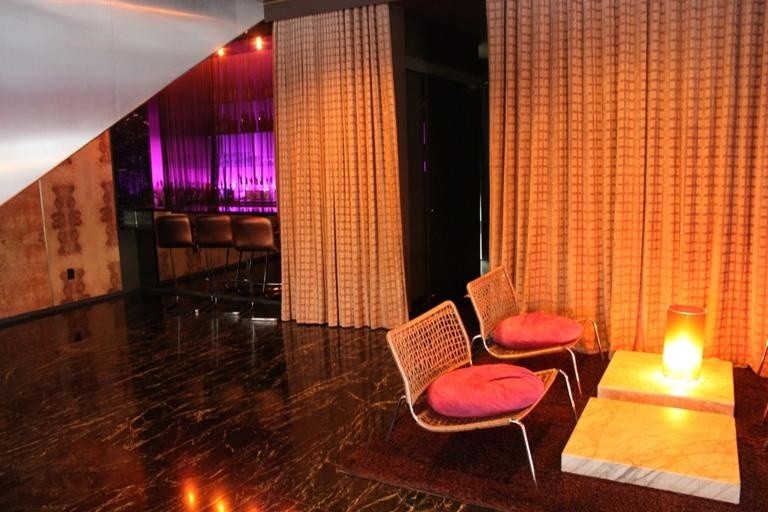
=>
[337,343,768,512]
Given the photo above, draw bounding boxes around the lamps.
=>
[663,304,706,381]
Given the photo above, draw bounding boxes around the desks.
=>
[561,397,742,506]
[597,350,734,415]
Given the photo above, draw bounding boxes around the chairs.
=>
[385,300,578,478]
[155,214,281,301]
[466,266,604,395]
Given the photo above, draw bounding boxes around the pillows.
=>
[427,364,545,418]
[492,311,583,347]
